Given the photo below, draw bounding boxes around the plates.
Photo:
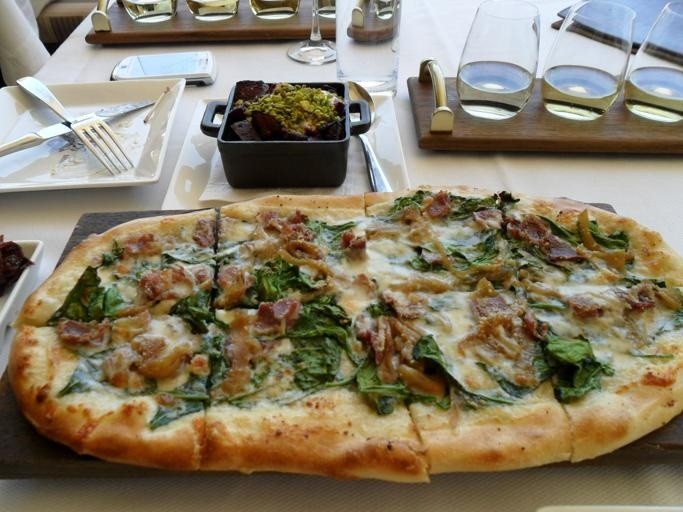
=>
[198,94,411,203]
[0,77,187,193]
[0,240,44,355]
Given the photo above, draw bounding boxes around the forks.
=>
[15,76,134,177]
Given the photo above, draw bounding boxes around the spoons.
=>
[346,81,392,192]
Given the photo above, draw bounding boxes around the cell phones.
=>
[110,50,218,88]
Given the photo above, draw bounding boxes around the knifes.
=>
[1,100,156,158]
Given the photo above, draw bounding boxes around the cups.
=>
[287,0,337,64]
[625,0,683,122]
[456,0,540,120]
[313,1,337,20]
[186,0,239,21]
[335,1,402,99]
[121,1,178,23]
[248,0,302,20]
[541,1,636,121]
